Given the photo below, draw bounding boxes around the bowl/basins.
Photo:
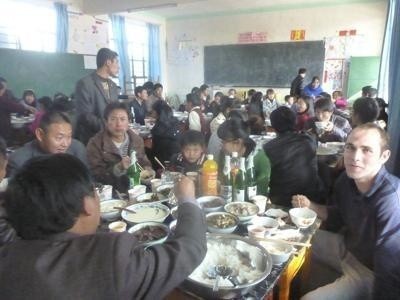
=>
[315,122,328,129]
[186,233,273,300]
[196,195,317,266]
[249,135,275,141]
[99,191,178,250]
[317,145,338,164]
[326,142,345,152]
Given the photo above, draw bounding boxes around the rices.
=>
[189,243,264,287]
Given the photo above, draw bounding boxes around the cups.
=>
[128,171,199,205]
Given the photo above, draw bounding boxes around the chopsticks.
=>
[137,162,150,178]
[297,194,303,214]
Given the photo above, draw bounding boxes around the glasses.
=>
[221,136,240,144]
[88,183,104,196]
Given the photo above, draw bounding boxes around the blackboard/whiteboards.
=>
[204,40,326,89]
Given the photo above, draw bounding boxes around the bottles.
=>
[127,149,141,189]
[203,151,257,203]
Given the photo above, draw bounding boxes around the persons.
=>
[1,47,399,299]
